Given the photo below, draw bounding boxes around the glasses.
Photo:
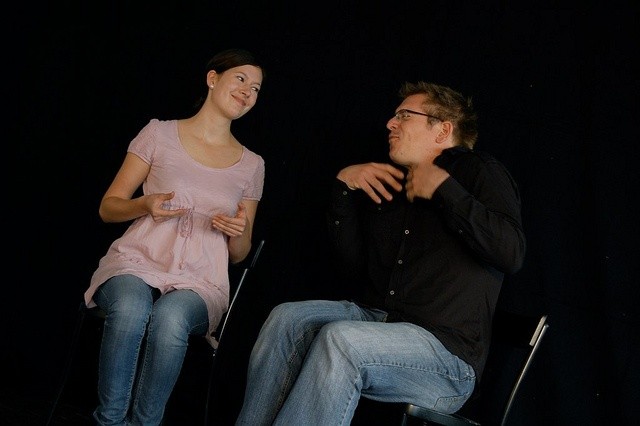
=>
[394,109,444,121]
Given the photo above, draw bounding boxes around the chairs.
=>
[45,230,263,423]
[390,304,555,424]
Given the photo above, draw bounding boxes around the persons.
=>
[234,82,524,426]
[84,48,265,426]
